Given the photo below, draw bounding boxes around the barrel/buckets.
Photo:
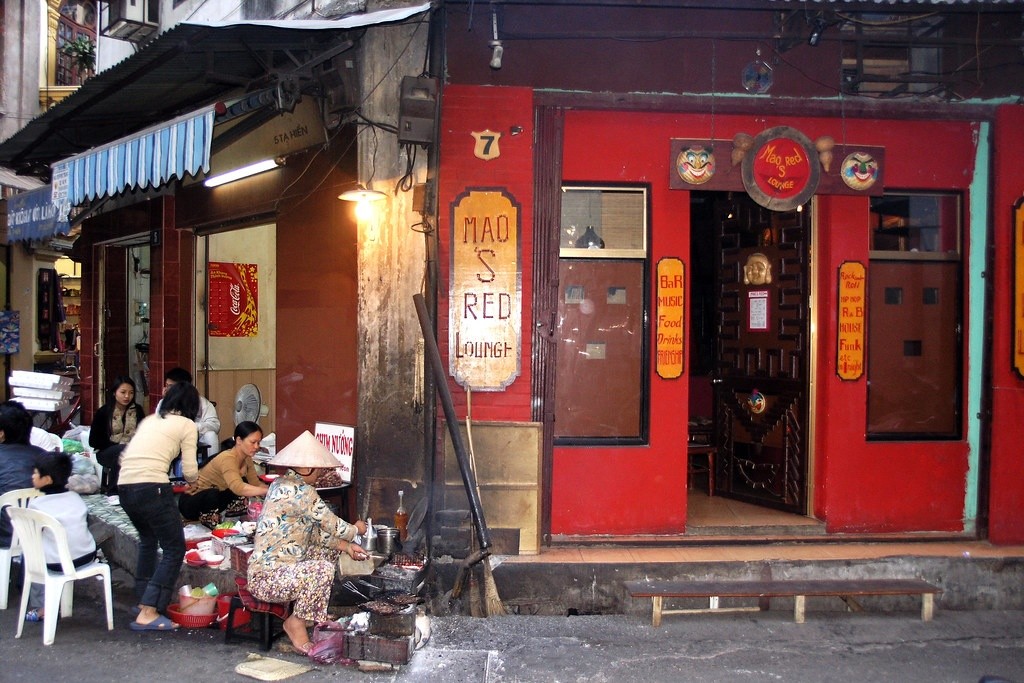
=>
[216,592,252,632]
[178,592,216,614]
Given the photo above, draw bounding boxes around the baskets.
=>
[168,604,219,627]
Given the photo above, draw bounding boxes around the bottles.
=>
[575,226,604,249]
[133,363,142,392]
[394,490,407,541]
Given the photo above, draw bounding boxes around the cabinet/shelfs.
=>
[58,276,81,349]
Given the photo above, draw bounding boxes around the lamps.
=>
[204,156,287,188]
[337,116,391,228]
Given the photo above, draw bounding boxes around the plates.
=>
[258,474,280,481]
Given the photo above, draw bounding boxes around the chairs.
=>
[0,487,50,610]
[6,505,112,645]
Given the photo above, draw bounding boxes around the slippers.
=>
[129,615,179,630]
[281,642,314,658]
[25,608,45,621]
[132,605,141,615]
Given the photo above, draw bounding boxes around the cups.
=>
[178,582,219,597]
[377,528,401,554]
[361,535,376,551]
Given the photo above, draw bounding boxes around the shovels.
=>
[359,580,422,605]
[342,581,410,616]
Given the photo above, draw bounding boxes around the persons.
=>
[117,380,200,631]
[88,376,146,495]
[247,430,370,656]
[0,400,48,548]
[154,366,220,476]
[178,421,268,522]
[24,452,97,622]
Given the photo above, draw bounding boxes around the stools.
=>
[225,594,286,653]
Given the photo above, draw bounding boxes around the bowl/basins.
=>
[185,552,207,566]
[185,537,212,551]
[211,529,240,539]
[205,554,225,569]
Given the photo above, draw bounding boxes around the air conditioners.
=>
[108,0,159,42]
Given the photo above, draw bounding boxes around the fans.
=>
[234,383,269,427]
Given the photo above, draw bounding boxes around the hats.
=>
[265,430,344,469]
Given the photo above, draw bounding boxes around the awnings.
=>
[0,2,431,244]
